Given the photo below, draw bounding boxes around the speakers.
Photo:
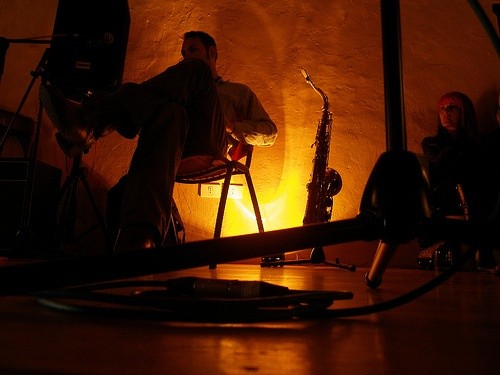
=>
[0,157,62,257]
[46,0,131,95]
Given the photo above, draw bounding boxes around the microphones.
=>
[65,33,114,44]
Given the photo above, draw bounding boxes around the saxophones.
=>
[299,67,343,222]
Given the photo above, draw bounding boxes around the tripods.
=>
[260,246,356,271]
[58,153,109,245]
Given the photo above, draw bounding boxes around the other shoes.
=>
[116,226,155,250]
[416,257,431,269]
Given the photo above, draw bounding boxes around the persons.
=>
[416,92,500,270]
[39,31,278,271]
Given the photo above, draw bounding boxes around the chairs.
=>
[112,145,264,269]
